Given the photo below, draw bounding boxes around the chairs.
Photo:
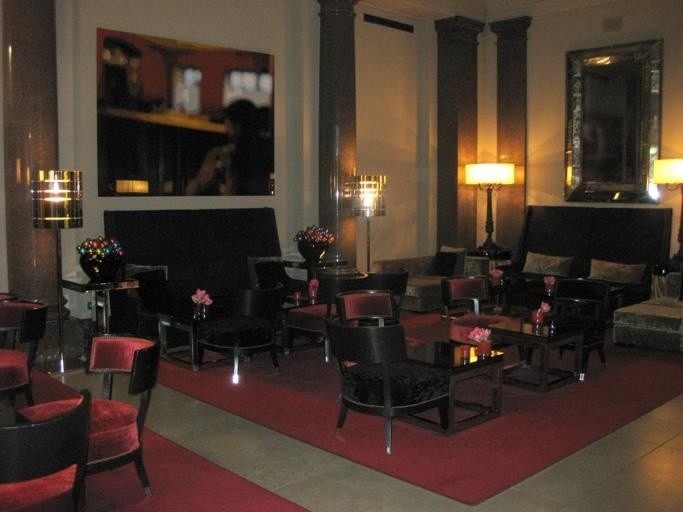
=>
[285,278,354,362]
[3,354,308,512]
[545,279,609,372]
[2,292,15,301]
[1,326,30,419]
[501,279,546,322]
[328,317,441,451]
[1,391,89,510]
[337,291,395,373]
[20,337,156,495]
[2,300,46,349]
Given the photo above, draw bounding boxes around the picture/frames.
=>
[565,40,664,205]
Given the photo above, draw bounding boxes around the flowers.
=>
[540,302,552,314]
[469,328,493,338]
[190,288,213,304]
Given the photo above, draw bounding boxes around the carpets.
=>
[153,339,613,505]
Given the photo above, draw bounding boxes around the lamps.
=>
[655,159,683,272]
[465,163,516,259]
[351,175,386,274]
[33,170,83,365]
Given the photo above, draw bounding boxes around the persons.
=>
[97,36,160,193]
[186,99,274,194]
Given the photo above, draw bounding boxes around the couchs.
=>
[496,205,672,328]
[104,208,308,349]
[373,255,491,312]
[614,273,683,351]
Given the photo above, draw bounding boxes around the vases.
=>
[479,340,492,355]
[298,241,328,265]
[194,304,207,320]
[533,311,544,334]
[80,253,117,280]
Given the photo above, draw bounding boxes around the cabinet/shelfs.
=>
[61,278,140,339]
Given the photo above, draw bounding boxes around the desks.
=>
[483,330,585,392]
[403,337,506,433]
[156,311,281,367]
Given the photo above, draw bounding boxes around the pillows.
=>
[589,259,645,285]
[433,251,457,277]
[521,252,575,279]
[249,256,307,301]
[125,264,168,314]
[440,245,468,278]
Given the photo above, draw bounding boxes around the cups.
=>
[294,291,301,302]
[307,279,320,299]
[475,342,491,357]
[532,310,544,330]
[461,345,470,358]
